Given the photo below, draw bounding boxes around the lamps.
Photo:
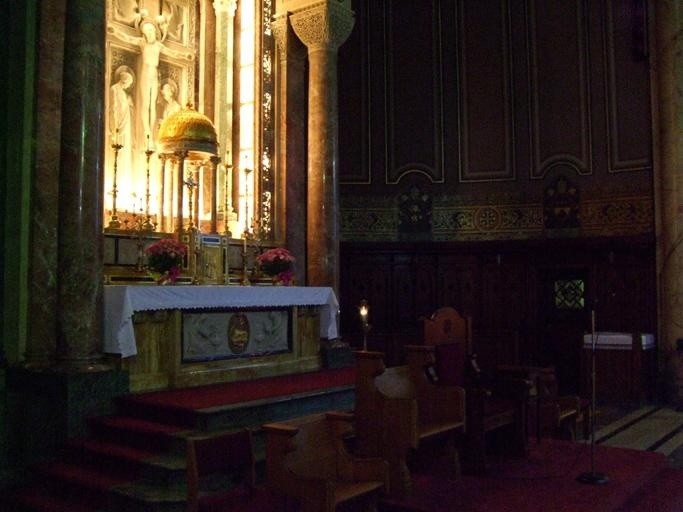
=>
[357,297,370,351]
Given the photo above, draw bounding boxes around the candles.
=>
[244,238,246,253]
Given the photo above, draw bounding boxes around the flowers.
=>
[143,235,188,285]
[256,247,297,286]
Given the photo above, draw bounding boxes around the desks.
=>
[582,332,655,407]
[103,287,341,394]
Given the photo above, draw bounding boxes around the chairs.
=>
[356,346,467,498]
[417,307,534,475]
[261,409,389,509]
[471,317,559,444]
[188,430,303,509]
